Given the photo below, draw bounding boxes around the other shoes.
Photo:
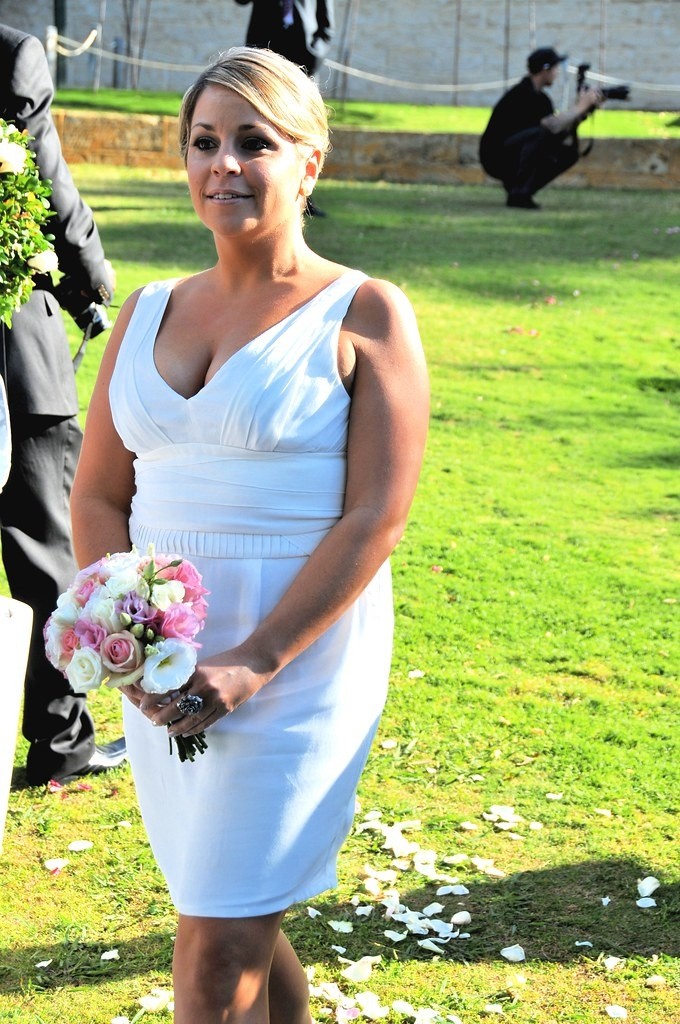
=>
[307,197,326,216]
[507,195,538,207]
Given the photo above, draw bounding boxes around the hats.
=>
[528,49,566,74]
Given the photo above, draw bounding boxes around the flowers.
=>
[0,114,66,329]
[44,541,209,763]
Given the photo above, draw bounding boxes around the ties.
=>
[283,0,294,25]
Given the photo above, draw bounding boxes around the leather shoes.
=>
[53,737,128,785]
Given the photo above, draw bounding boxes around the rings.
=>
[177,694,202,716]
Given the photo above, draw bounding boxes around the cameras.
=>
[577,63,630,113]
[53,273,112,339]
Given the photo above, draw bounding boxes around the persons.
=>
[0,22,132,780]
[235,0,336,218]
[72,45,432,1024]
[479,47,606,209]
